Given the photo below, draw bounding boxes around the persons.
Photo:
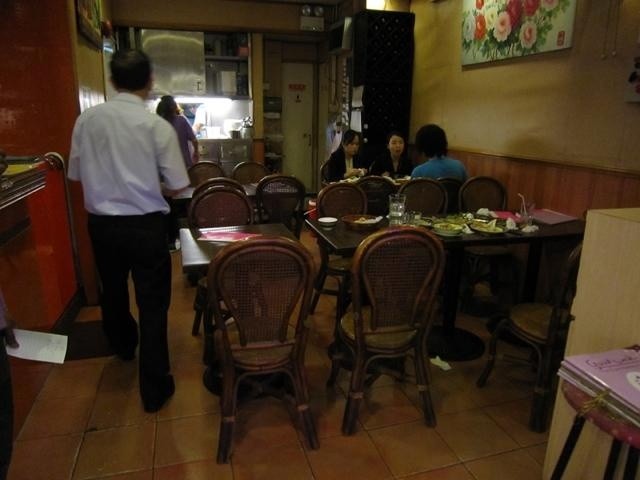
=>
[409,123,466,182]
[156,94,200,254]
[321,130,369,183]
[65,48,193,414]
[371,132,414,179]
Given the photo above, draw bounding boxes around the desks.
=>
[169,181,317,220]
[302,207,587,363]
[180,222,300,394]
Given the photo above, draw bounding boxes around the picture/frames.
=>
[75,0,103,50]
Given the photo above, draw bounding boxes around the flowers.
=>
[461,0,566,61]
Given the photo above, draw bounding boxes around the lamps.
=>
[299,5,325,32]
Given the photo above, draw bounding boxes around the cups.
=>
[389,192,408,217]
[520,201,536,227]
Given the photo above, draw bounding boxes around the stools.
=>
[553,377,638,479]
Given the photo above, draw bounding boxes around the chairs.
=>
[481,240,586,433]
[328,228,445,438]
[189,158,509,337]
[207,235,317,463]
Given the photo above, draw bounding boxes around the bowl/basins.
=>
[342,214,382,230]
[318,217,338,227]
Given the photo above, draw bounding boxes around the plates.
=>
[410,208,509,236]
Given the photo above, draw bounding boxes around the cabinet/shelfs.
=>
[130,26,252,104]
[347,8,416,159]
[539,207,640,480]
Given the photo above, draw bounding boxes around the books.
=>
[557,349,640,431]
